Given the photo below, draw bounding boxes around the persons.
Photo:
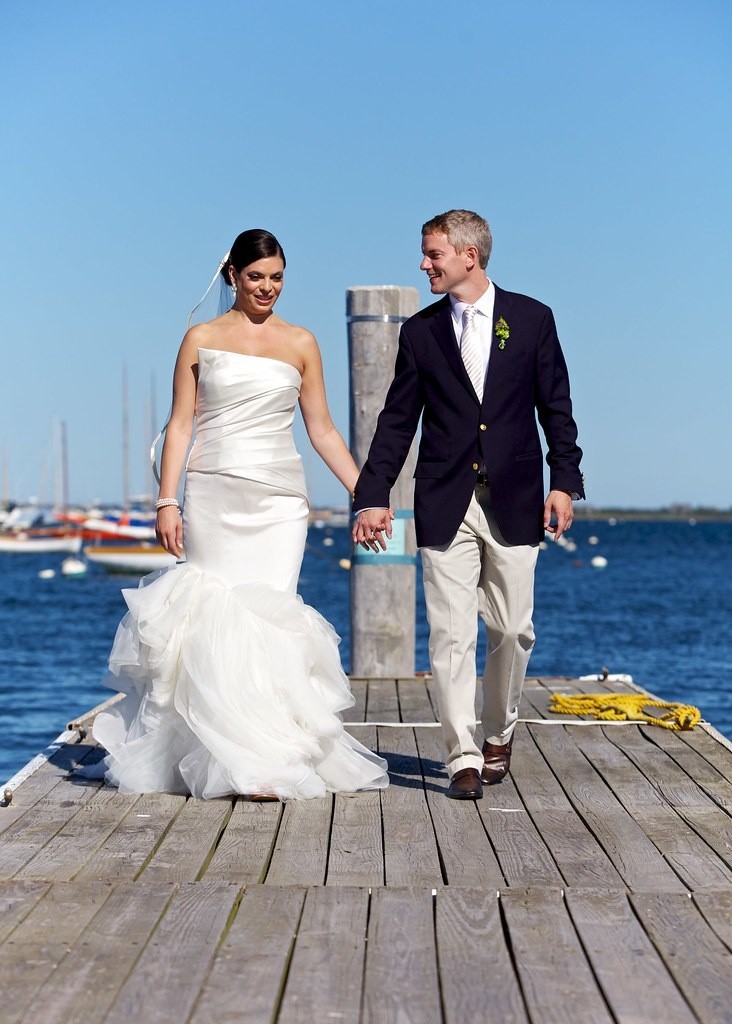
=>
[353,210,585,798]
[73,229,389,803]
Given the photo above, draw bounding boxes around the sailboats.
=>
[0,360,188,576]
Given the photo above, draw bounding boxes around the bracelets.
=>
[155,498,178,510]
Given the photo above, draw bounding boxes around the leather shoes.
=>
[481,731,514,785]
[447,767,484,799]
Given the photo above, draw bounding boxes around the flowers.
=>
[495,316,510,350]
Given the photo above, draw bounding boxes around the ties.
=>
[460,307,485,405]
